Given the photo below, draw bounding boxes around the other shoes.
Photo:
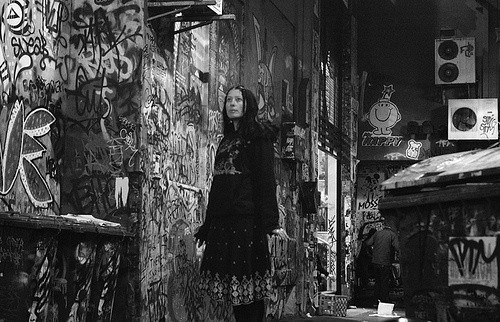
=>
[355,300,368,308]
[371,301,378,308]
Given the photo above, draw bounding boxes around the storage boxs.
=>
[320,294,348,317]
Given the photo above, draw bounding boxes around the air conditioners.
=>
[152,0,222,17]
[435,38,476,84]
[447,98,498,140]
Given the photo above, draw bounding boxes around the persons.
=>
[193,83,290,321]
[354,228,378,308]
[366,217,401,309]
